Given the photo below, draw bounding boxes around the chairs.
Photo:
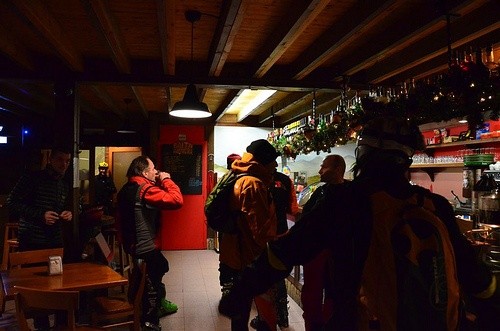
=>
[0,223,148,331]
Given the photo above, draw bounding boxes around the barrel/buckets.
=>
[462,154,494,198]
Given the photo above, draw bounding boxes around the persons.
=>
[94,161,116,216]
[216,112,497,331]
[7,145,75,331]
[118,155,184,315]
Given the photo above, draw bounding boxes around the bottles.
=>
[471,174,500,224]
[293,175,305,193]
[274,42,500,140]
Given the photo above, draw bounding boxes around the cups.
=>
[155,172,160,179]
[411,147,500,162]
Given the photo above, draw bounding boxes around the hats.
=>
[99,162,108,168]
[227,154,242,164]
[246,140,278,164]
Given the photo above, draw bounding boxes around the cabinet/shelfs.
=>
[409,136,500,168]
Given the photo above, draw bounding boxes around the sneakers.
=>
[159,299,177,314]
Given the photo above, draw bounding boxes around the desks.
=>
[0,262,129,331]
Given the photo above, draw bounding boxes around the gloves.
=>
[219,282,257,318]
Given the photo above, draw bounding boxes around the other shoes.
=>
[251,317,275,331]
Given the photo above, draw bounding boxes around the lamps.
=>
[168,9,212,119]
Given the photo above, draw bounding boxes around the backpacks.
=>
[205,173,255,232]
